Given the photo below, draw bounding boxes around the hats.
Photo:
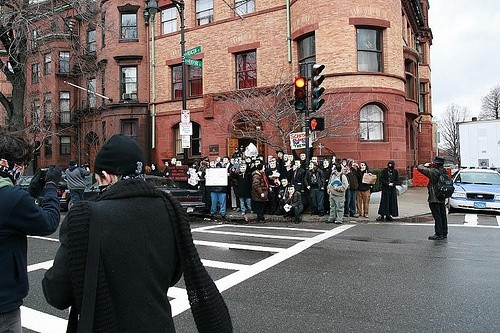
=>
[431,156,445,164]
[93,134,146,176]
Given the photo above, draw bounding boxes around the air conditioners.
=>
[123,92,132,100]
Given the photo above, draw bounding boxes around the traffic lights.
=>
[311,62,325,111]
[309,117,325,131]
[295,76,306,111]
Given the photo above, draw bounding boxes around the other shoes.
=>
[349,214,358,217]
[385,216,393,222]
[344,213,349,217]
[258,218,265,223]
[335,220,342,224]
[311,210,324,216]
[356,213,362,216]
[362,213,368,217]
[376,216,385,222]
[325,220,333,223]
[209,207,253,218]
[294,217,299,224]
[429,234,447,240]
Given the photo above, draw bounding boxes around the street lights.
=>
[146,1,190,165]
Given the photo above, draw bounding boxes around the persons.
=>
[232,145,245,158]
[65,161,90,202]
[417,157,449,239]
[193,149,373,224]
[42,134,234,333]
[0,125,62,333]
[376,161,399,221]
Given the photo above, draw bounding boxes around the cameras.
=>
[41,168,55,179]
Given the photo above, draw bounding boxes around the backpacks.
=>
[431,168,454,198]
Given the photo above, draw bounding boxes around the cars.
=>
[447,169,500,212]
[16,172,206,219]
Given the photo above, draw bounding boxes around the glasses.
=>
[23,160,29,169]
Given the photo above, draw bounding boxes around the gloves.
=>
[28,168,47,199]
[45,165,62,186]
